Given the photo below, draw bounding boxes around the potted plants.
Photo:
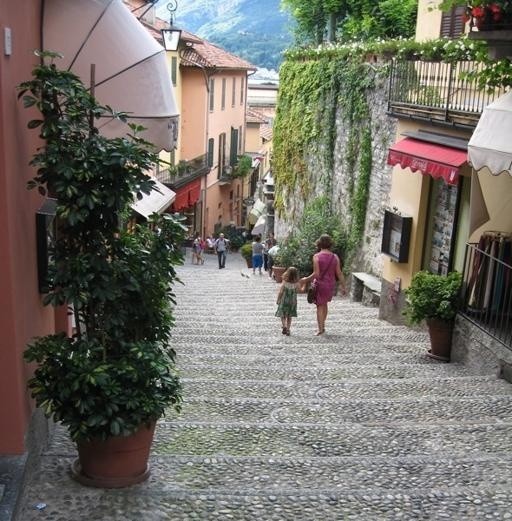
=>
[238,245,252,270]
[8,47,192,492]
[400,269,461,361]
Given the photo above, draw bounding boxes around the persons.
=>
[191,230,231,269]
[274,267,307,336]
[251,232,279,277]
[302,234,347,335]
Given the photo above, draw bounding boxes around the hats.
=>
[219,233,224,237]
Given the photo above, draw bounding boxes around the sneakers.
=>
[219,265,225,269]
[282,328,290,335]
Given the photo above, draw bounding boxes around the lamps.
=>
[130,0,182,53]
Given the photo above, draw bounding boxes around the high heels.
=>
[318,329,325,335]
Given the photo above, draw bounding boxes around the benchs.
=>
[348,271,380,306]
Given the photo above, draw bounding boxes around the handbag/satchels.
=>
[307,286,315,303]
